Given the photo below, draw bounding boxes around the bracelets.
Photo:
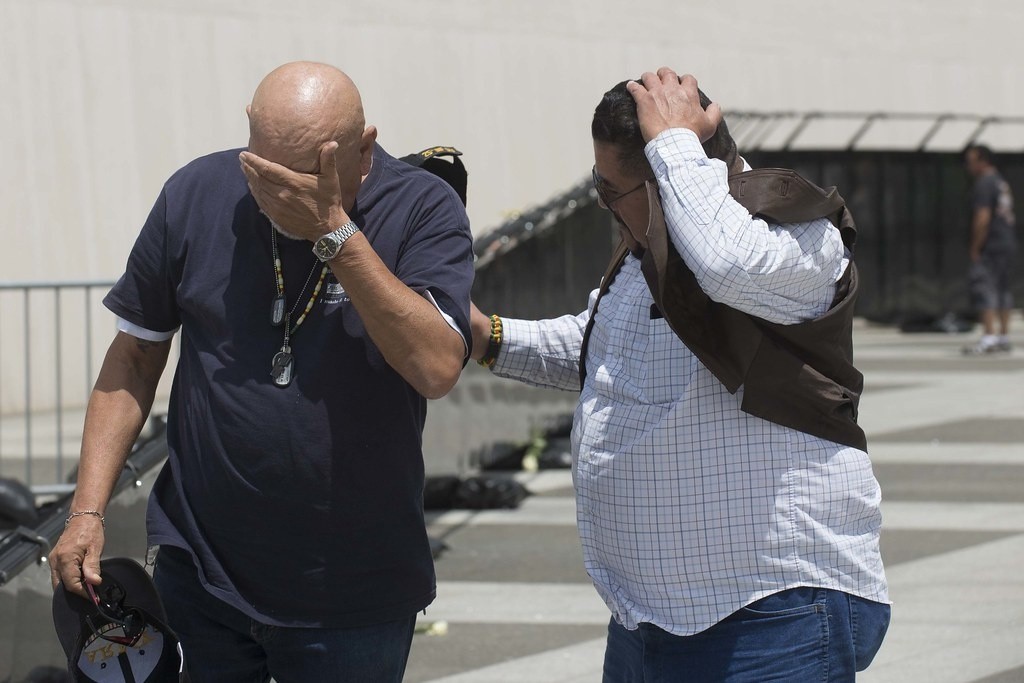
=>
[65,510,105,530]
[477,315,502,367]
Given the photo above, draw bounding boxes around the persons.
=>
[48,61,474,683]
[966,146,1016,355]
[470,67,892,683]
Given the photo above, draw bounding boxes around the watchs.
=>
[312,221,359,263]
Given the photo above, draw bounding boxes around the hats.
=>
[397,146,468,210]
[52,557,184,683]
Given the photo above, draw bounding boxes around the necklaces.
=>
[272,225,329,388]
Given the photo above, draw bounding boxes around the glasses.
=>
[83,571,146,648]
[593,165,656,216]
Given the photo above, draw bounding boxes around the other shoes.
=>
[960,339,1014,357]
